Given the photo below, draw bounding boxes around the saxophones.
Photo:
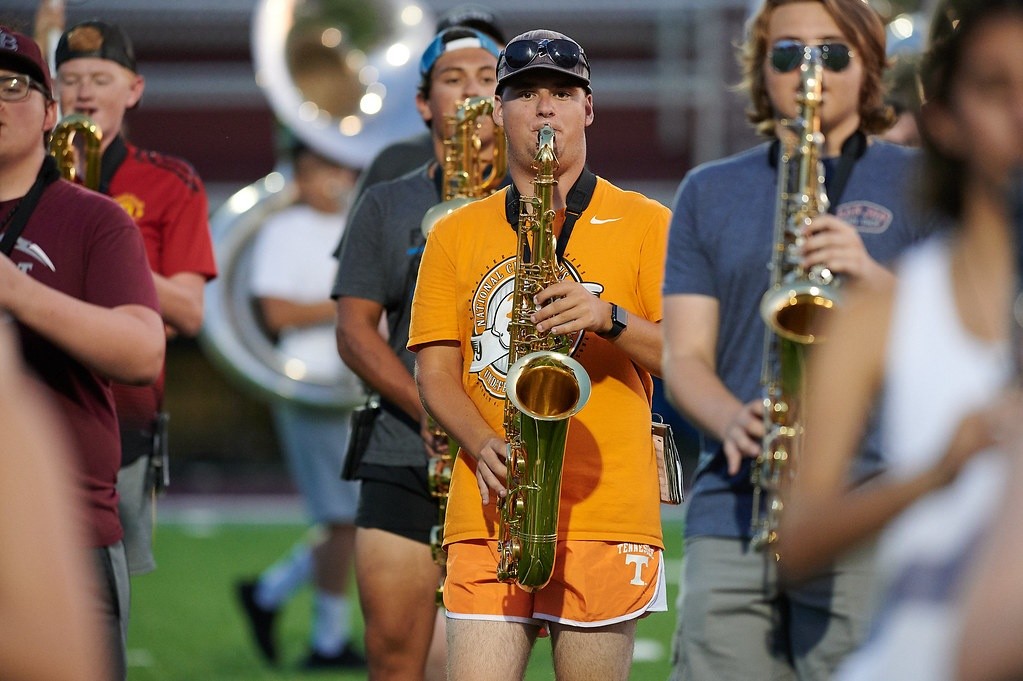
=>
[750,47,835,552]
[421,93,505,241]
[500,123,591,592]
[49,118,104,188]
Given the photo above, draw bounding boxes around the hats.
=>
[55,19,137,73]
[418,25,500,74]
[494,29,591,86]
[0,24,52,101]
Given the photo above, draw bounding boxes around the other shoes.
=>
[304,639,364,667]
[234,576,279,663]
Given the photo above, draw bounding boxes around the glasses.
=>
[0,74,54,101]
[767,38,852,75]
[496,38,591,78]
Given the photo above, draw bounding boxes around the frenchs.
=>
[197,2,453,413]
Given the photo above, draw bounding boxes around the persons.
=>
[661,1,922,681]
[53,18,219,339]
[773,0,1023,681]
[405,29,674,681]
[235,142,362,672]
[331,17,506,261]
[0,25,167,681]
[329,26,500,681]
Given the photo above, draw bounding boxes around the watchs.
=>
[593,302,628,340]
